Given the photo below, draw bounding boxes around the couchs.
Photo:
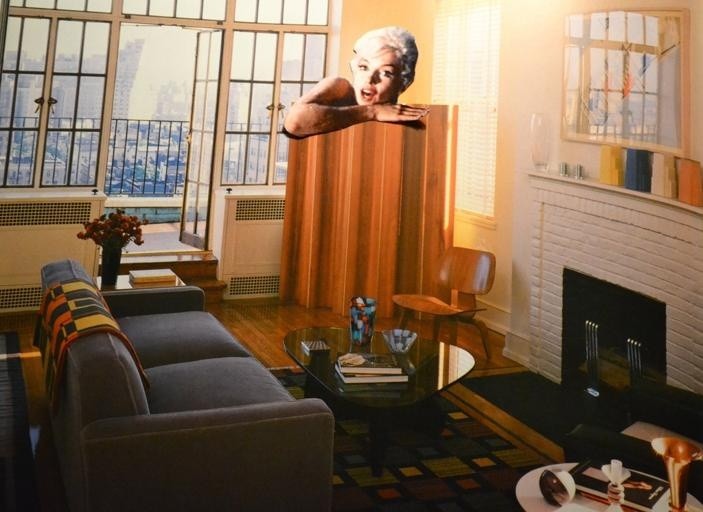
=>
[41,258,335,511]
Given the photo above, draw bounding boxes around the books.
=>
[129,268,176,284]
[575,488,642,512]
[568,457,671,512]
[336,351,402,374]
[333,359,408,382]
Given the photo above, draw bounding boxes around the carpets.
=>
[0,331,42,511]
[266,363,564,511]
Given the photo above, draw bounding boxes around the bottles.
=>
[528,111,554,173]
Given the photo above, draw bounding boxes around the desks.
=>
[93,275,186,292]
[283,326,475,442]
[515,463,703,512]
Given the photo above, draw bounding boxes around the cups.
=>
[348,296,376,345]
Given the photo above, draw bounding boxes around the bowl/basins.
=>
[382,328,418,354]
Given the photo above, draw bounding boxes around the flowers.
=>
[77,209,145,246]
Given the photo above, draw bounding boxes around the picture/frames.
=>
[560,7,691,159]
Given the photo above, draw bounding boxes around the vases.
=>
[101,245,122,285]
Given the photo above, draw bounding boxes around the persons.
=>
[282,26,429,140]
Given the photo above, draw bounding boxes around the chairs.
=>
[392,246,495,359]
